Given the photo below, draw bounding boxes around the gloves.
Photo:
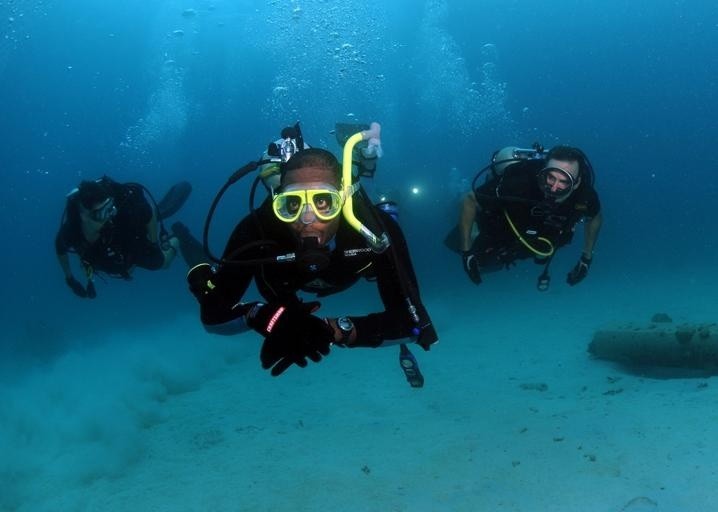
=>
[461,249,483,285]
[247,300,335,377]
[72,282,88,298]
[567,254,592,285]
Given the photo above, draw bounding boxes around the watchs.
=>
[336,316,353,344]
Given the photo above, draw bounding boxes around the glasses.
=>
[270,182,344,224]
[93,198,116,222]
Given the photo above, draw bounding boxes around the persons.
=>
[55,177,181,298]
[461,145,603,285]
[171,148,439,377]
